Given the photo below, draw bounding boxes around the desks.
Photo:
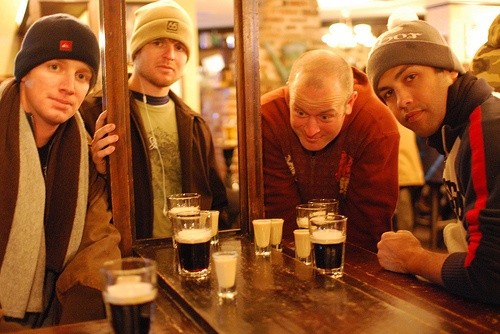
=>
[135,233,500,334]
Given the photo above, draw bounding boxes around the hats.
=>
[366,20,466,106]
[129,0,194,64]
[14,13,100,98]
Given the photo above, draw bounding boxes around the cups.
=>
[271,218,284,252]
[309,215,348,279]
[307,198,338,220]
[166,192,202,248]
[295,203,327,270]
[99,257,158,334]
[200,210,220,245]
[212,251,238,298]
[293,229,313,266]
[171,210,213,279]
[251,219,272,255]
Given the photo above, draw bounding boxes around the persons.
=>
[90,0,232,240]
[366,21,500,315]
[1,13,122,332]
[394,117,425,230]
[260,48,400,253]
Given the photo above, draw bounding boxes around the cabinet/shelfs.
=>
[198,27,236,50]
